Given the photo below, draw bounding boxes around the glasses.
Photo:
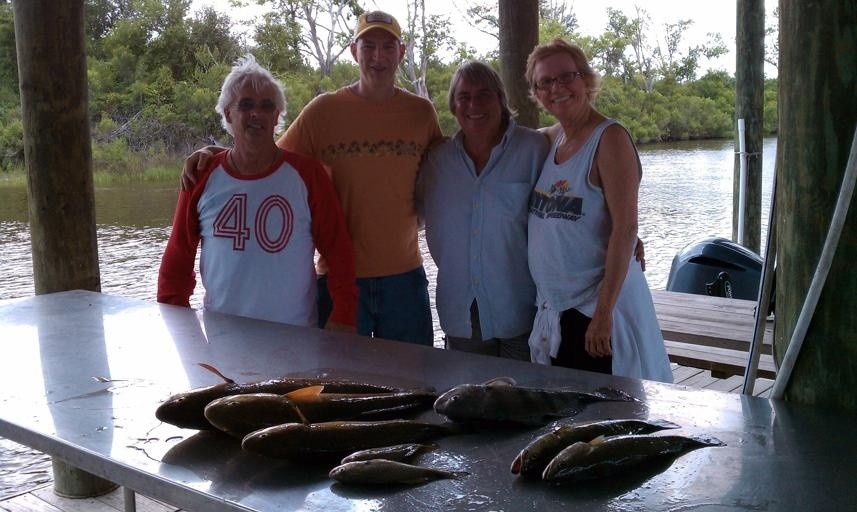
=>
[230,100,275,113]
[534,71,585,90]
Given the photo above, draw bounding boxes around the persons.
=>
[415,59,551,363]
[155,50,357,332]
[273,8,445,346]
[525,36,674,386]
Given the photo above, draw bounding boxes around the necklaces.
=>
[229,140,279,175]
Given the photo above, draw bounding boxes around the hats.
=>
[355,11,401,45]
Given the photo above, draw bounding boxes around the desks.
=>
[0,289,857,512]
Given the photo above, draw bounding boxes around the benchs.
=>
[650,290,779,379]
